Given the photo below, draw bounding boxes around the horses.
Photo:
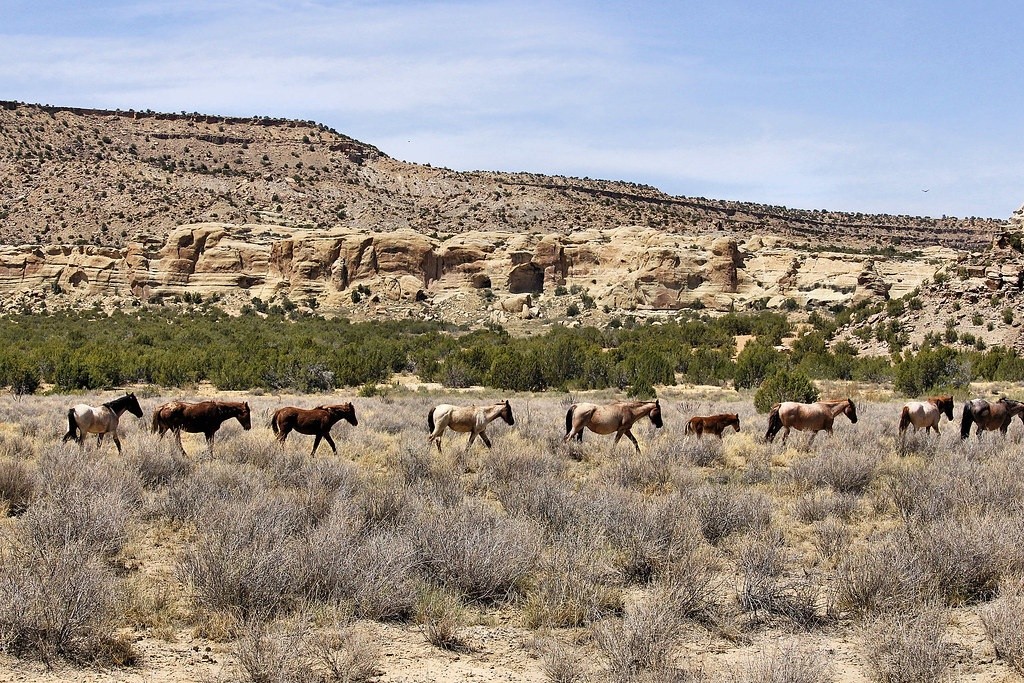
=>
[151,399,251,458]
[684,412,741,442]
[272,401,358,458]
[898,395,955,438]
[564,398,664,456]
[961,397,1024,439]
[765,398,858,452]
[61,392,144,456]
[428,399,515,454]
